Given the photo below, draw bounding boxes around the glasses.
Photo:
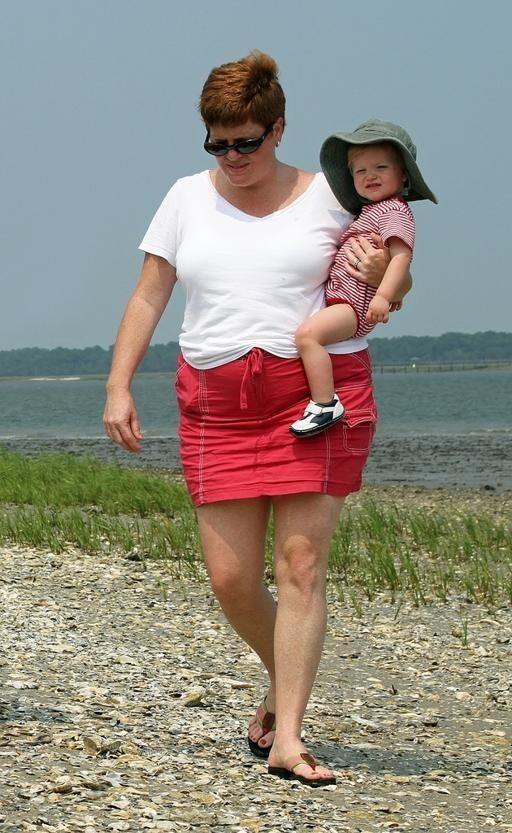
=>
[204,126,271,157]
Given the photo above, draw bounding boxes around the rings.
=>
[353,259,362,269]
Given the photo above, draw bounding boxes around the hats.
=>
[320,117,438,215]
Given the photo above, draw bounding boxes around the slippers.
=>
[248,695,274,758]
[269,751,338,786]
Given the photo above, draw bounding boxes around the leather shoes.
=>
[289,392,345,438]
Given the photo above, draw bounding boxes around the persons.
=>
[101,48,414,784]
[289,119,417,437]
